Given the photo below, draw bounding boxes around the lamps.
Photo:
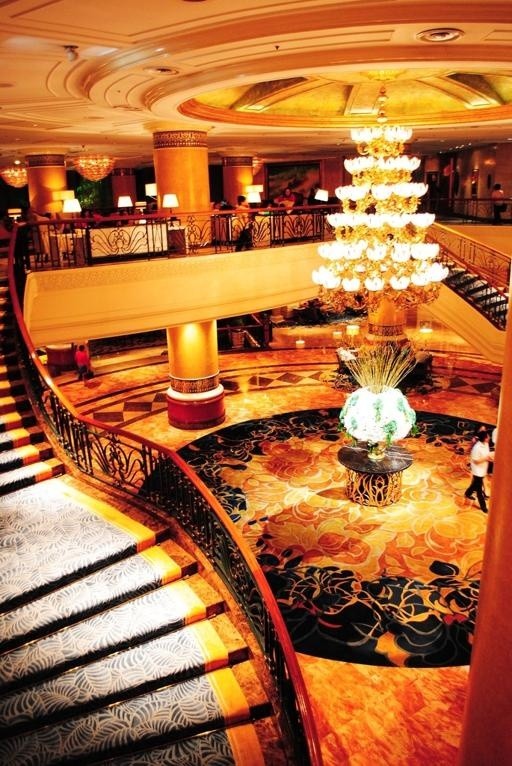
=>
[311,79,448,307]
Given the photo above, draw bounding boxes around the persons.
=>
[465,425,495,513]
[73,344,95,388]
[48,188,299,251]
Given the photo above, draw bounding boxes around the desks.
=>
[336,443,412,508]
